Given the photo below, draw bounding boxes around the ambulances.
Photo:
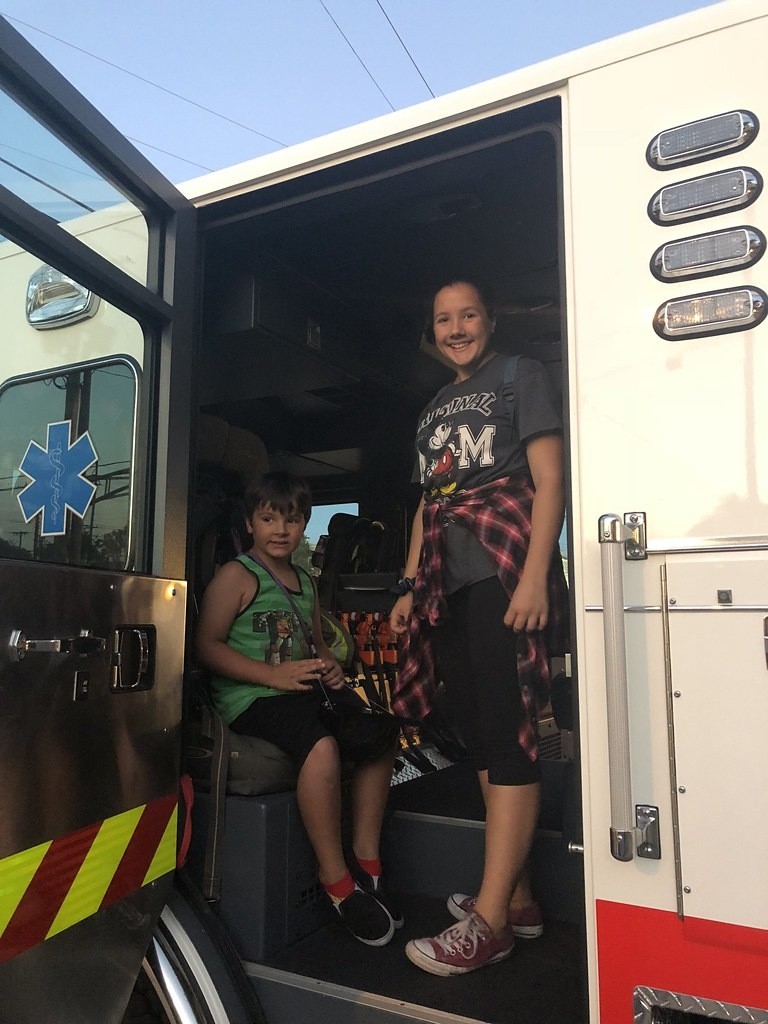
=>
[0,1,767,1024]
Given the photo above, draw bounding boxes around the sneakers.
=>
[446,892,544,939]
[404,908,516,976]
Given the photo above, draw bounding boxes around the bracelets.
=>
[398,576,415,596]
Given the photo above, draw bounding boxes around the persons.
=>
[193,471,404,946]
[389,267,567,977]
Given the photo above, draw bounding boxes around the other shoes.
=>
[315,875,395,946]
[343,847,405,929]
